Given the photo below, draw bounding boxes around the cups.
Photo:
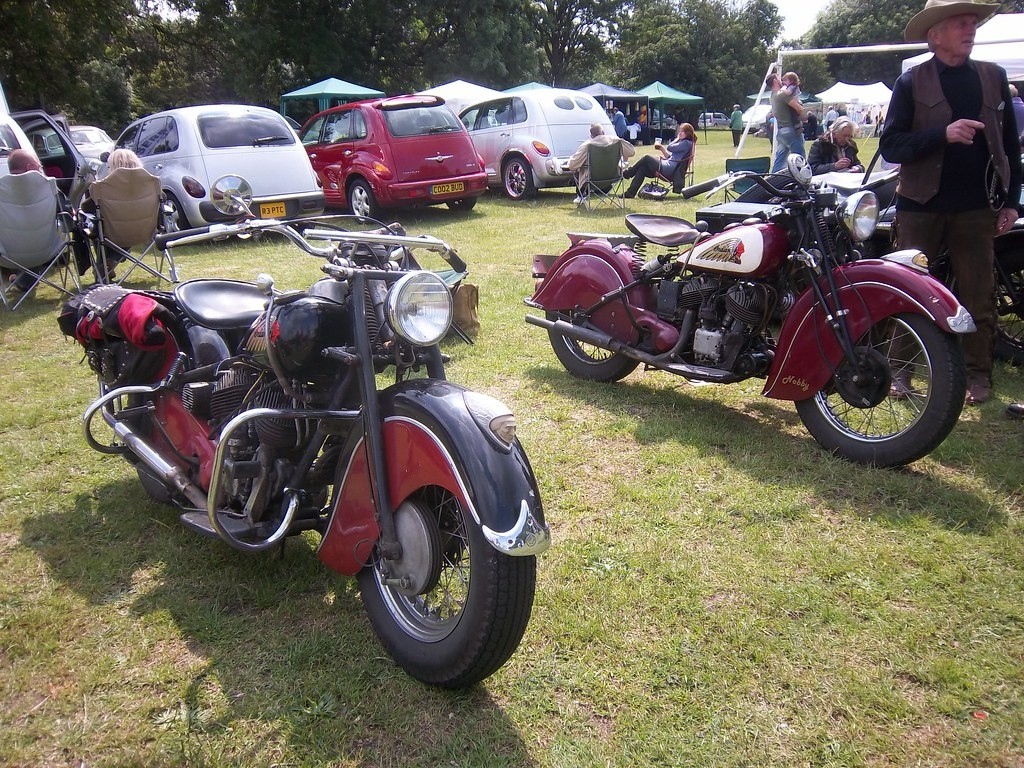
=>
[655,138,662,146]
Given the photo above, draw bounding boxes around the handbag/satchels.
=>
[447,284,480,337]
[637,181,669,201]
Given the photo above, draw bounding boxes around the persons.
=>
[611,107,627,138]
[568,123,635,203]
[879,0,1023,404]
[1009,84,1024,153]
[863,110,871,125]
[81,148,143,280]
[729,104,743,147]
[617,123,694,198]
[807,110,817,140]
[766,72,807,175]
[807,115,865,177]
[824,106,837,132]
[7,149,75,291]
[875,111,883,135]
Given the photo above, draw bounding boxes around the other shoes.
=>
[619,166,630,180]
[888,370,913,399]
[795,128,805,137]
[9,273,40,292]
[573,196,587,203]
[965,383,991,405]
[615,192,633,198]
[553,157,563,176]
[95,259,117,283]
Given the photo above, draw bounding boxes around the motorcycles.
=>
[517,152,978,471]
[58,175,553,693]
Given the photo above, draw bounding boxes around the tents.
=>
[815,82,893,121]
[279,78,386,115]
[578,82,649,144]
[636,81,707,145]
[902,13,1024,82]
[418,79,551,113]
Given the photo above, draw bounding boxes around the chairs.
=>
[76,167,177,288]
[0,171,81,312]
[724,156,771,204]
[571,141,627,212]
[655,153,696,189]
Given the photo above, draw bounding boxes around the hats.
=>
[904,0,1001,43]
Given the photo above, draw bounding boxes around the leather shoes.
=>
[1006,402,1024,418]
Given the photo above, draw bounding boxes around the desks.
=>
[652,128,676,143]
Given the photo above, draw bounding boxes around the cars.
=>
[455,87,621,201]
[295,92,491,226]
[112,102,326,246]
[0,108,93,206]
[649,108,673,126]
[30,126,121,169]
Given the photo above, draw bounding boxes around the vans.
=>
[740,105,773,134]
[698,112,732,128]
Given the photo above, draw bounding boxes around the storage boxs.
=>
[697,201,781,235]
